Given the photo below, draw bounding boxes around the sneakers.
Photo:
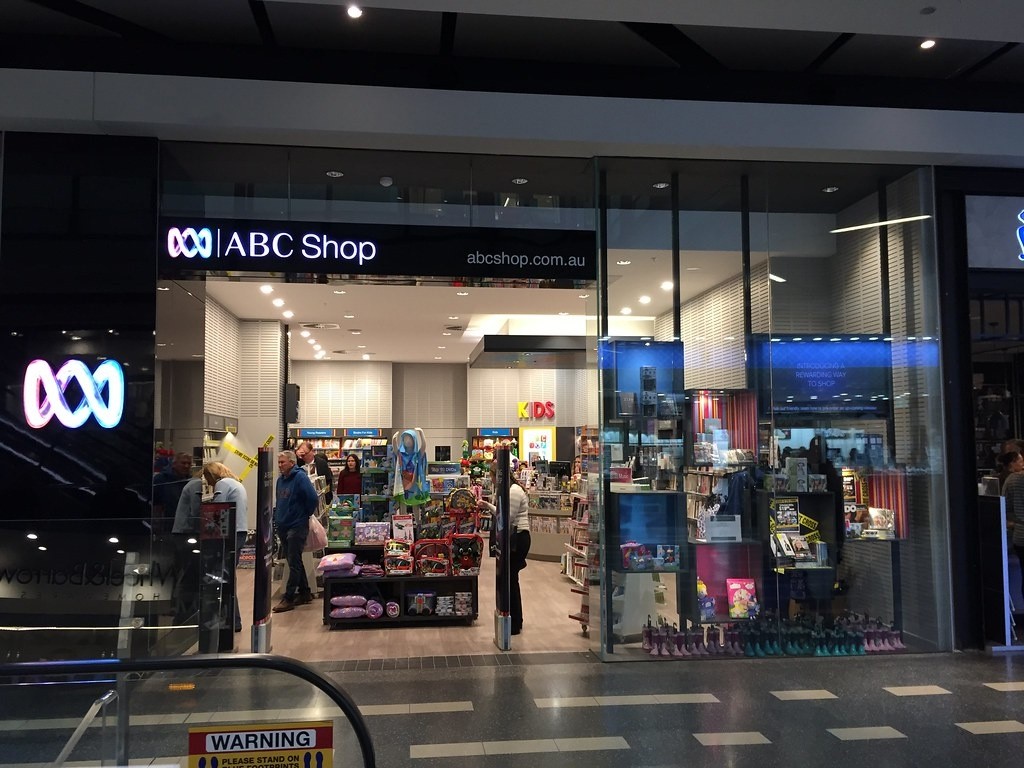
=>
[293,590,312,604]
[272,594,294,612]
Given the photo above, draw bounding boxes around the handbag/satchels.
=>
[303,515,328,552]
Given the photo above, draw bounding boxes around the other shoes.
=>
[235,618,242,631]
[511,619,523,635]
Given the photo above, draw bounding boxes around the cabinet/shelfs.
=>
[574,427,600,480]
[659,460,757,546]
[321,541,479,632]
[563,492,603,588]
[286,435,389,493]
[468,428,519,537]
[277,465,330,599]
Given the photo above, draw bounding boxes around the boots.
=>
[643,611,906,658]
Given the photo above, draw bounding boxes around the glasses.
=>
[300,450,312,458]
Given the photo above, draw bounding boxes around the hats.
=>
[490,460,514,472]
[314,454,328,464]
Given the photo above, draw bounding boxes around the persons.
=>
[203,461,248,631]
[874,509,893,529]
[999,439,1024,598]
[512,459,518,471]
[172,469,203,626]
[477,460,531,635]
[573,455,581,474]
[697,580,715,619]
[392,427,431,524]
[775,462,824,492]
[745,596,759,620]
[296,442,333,506]
[784,539,808,558]
[336,454,362,494]
[272,451,319,611]
